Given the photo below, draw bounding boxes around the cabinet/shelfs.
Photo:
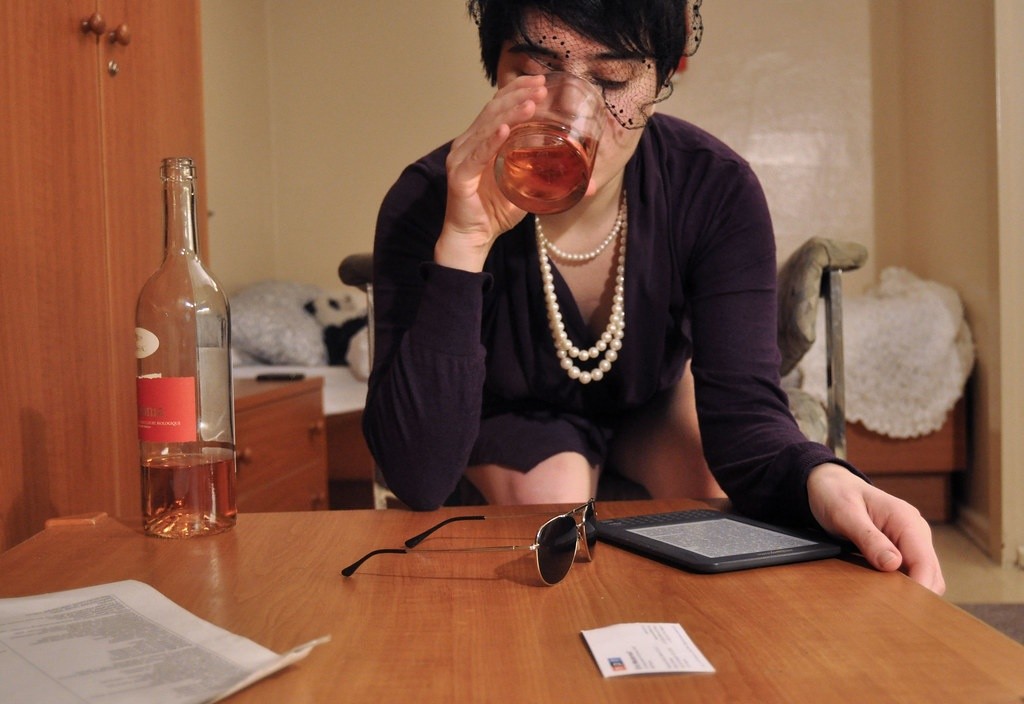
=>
[232,377,326,513]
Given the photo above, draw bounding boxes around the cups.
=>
[493,72,608,213]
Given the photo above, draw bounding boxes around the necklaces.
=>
[535,190,628,384]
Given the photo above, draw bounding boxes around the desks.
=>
[0,496,1024,704]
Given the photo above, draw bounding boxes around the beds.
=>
[227,264,981,524]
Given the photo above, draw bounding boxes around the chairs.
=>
[339,237,868,508]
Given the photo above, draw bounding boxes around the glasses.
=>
[343,497,598,586]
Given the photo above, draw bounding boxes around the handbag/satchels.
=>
[777,235,868,374]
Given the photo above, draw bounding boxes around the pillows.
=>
[227,278,332,367]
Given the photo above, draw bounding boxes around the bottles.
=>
[135,156,240,539]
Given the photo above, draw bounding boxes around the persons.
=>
[361,0,947,600]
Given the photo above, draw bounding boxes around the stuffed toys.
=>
[306,294,367,367]
